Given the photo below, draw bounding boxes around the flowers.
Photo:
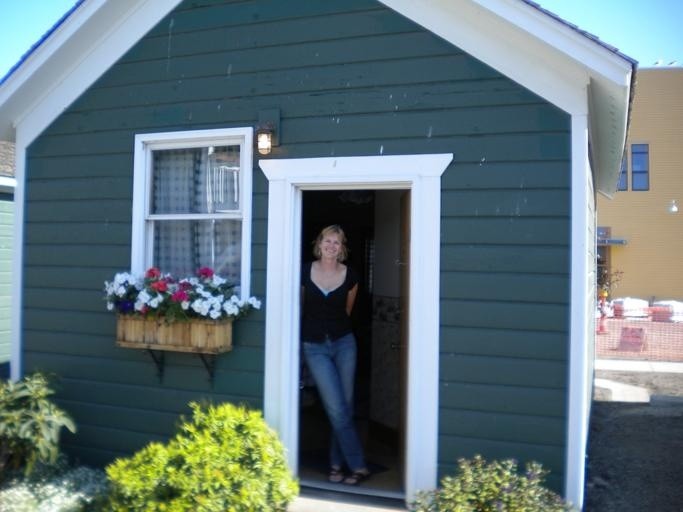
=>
[100,261,263,321]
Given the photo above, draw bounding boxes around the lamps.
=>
[254,124,277,155]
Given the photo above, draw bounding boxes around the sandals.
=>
[342,470,374,485]
[327,465,345,483]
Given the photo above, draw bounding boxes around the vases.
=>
[114,312,234,355]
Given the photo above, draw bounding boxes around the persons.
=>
[300,223,371,487]
